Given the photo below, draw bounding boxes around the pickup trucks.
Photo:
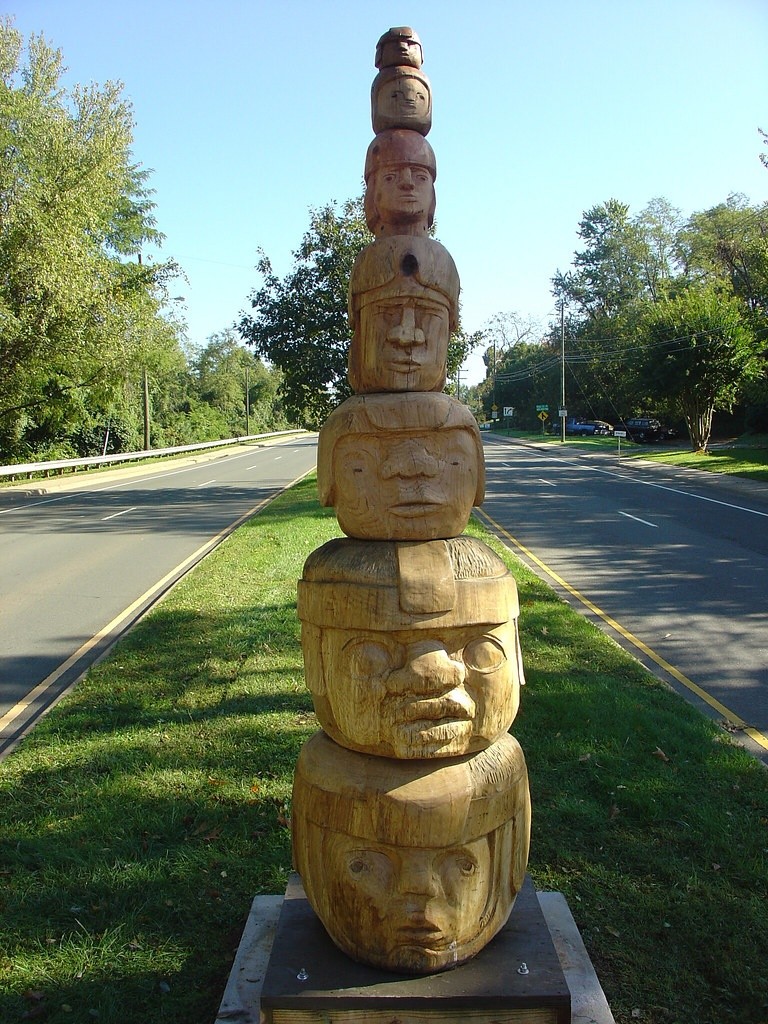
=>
[553,417,596,437]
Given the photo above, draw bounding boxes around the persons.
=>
[292,27,532,968]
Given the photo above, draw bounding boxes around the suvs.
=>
[613,417,663,442]
